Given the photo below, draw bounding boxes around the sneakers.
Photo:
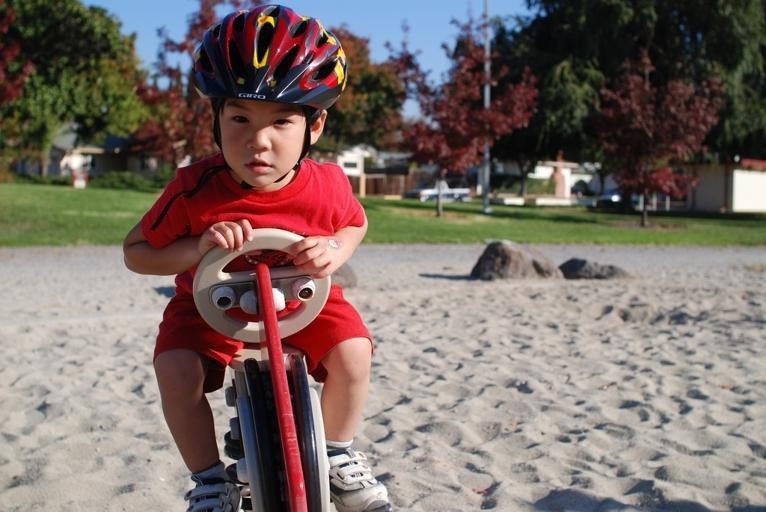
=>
[322,440,391,512]
[183,460,244,512]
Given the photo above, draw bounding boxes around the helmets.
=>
[192,3,349,112]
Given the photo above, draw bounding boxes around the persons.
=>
[119,3,393,511]
